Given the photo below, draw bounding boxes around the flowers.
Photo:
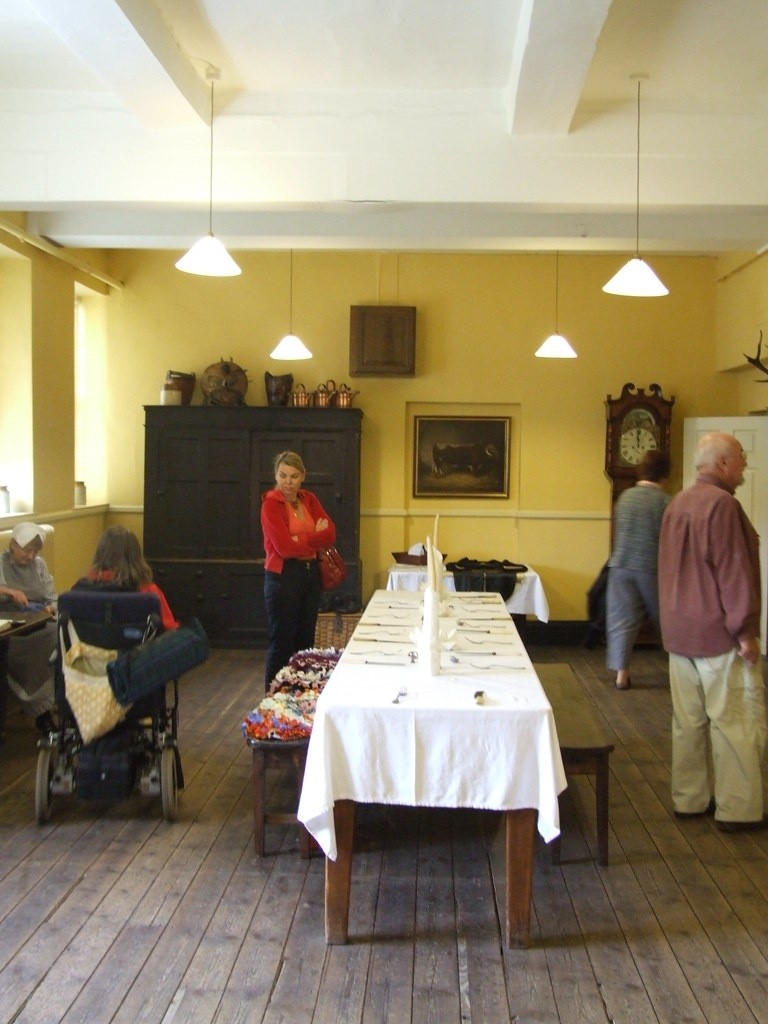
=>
[241,648,340,740]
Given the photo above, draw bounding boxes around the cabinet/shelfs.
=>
[143,404,365,652]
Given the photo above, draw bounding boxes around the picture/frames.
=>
[349,303,417,380]
[413,415,513,501]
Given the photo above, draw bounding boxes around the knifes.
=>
[347,591,526,704]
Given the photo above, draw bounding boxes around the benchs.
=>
[533,663,614,870]
[243,645,343,858]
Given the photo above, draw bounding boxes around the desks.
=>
[0,610,54,744]
[297,590,569,949]
[388,562,550,644]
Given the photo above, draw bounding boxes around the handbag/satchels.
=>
[315,543,348,590]
[57,619,135,747]
[318,591,360,614]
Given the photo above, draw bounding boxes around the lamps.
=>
[532,249,578,361]
[602,72,672,296]
[173,68,242,278]
[271,249,314,364]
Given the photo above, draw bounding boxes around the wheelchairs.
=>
[34,587,185,823]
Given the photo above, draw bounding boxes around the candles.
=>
[433,514,440,549]
[427,537,437,591]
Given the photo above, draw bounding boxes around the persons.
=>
[605,450,687,689]
[0,524,60,740]
[260,452,336,695]
[75,523,181,632]
[656,431,768,831]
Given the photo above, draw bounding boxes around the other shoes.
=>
[715,813,768,833]
[35,711,54,740]
[675,799,716,819]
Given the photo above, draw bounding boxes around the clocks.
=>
[602,381,675,548]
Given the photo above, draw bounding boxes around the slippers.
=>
[615,673,631,690]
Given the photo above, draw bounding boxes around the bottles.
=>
[160,385,182,405]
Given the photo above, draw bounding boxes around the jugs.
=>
[293,380,359,409]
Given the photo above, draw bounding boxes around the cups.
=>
[264,372,293,408]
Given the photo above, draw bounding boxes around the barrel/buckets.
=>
[165,369,195,406]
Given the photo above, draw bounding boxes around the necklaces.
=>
[287,499,298,516]
